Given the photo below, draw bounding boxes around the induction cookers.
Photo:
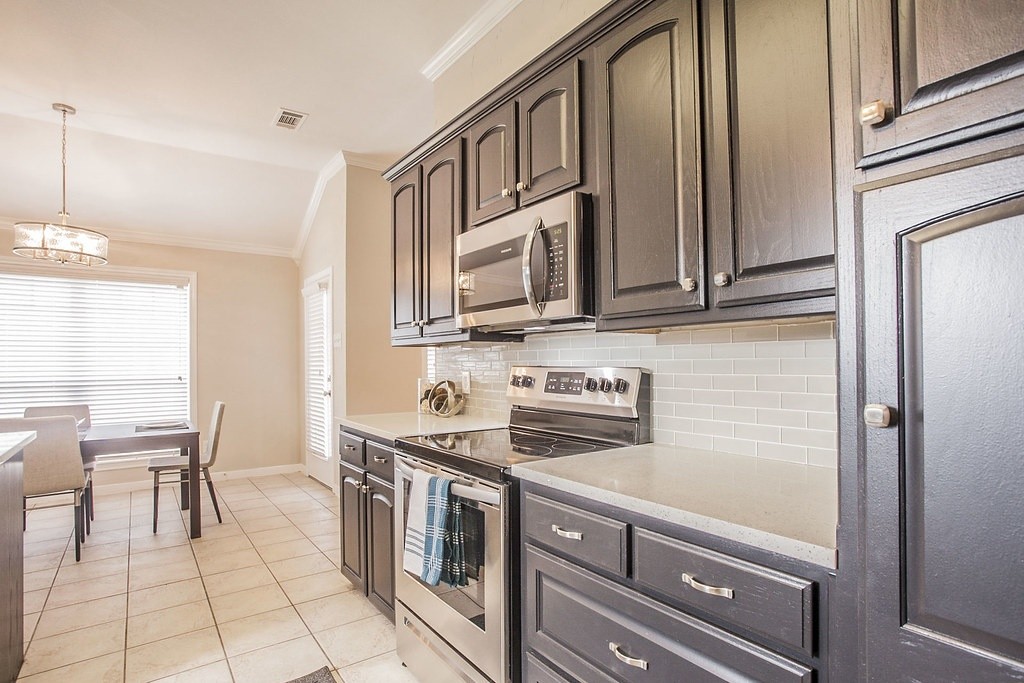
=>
[394,366,650,484]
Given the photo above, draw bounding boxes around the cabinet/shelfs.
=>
[589,0,838,333]
[389,134,524,347]
[338,424,396,625]
[464,46,591,228]
[519,477,833,683]
[0,430,38,683]
[830,0,1024,683]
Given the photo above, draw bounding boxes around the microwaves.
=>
[456,191,595,335]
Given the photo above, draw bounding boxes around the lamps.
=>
[13,103,110,267]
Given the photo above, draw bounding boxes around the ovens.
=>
[394,454,520,683]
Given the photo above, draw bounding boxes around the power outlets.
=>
[461,371,471,394]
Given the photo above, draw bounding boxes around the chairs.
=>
[0,415,90,563]
[147,401,225,533]
[24,404,96,536]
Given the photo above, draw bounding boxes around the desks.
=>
[77,420,202,540]
